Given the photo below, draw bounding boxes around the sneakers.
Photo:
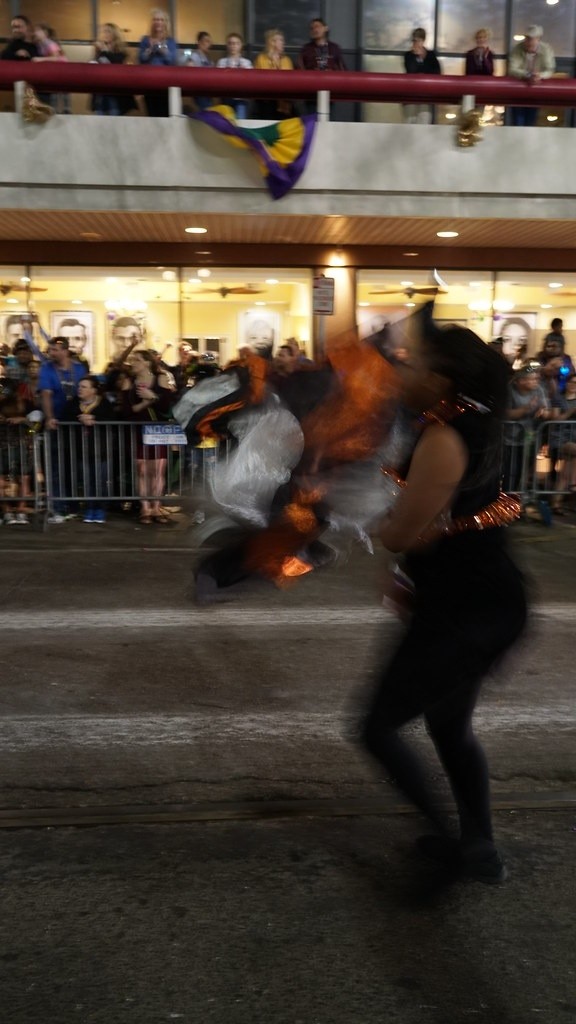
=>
[83,510,94,523]
[94,509,106,523]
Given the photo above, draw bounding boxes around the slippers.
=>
[139,511,154,527]
[153,511,169,525]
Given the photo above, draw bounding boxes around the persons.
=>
[466,29,495,120]
[5,315,38,354]
[186,31,216,112]
[0,318,576,883]
[498,316,532,356]
[403,27,441,125]
[244,319,274,350]
[57,319,86,356]
[139,11,177,117]
[112,317,142,356]
[297,17,347,117]
[217,33,253,119]
[0,15,73,114]
[92,22,140,116]
[506,25,556,126]
[253,28,294,120]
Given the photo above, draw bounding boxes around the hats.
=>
[524,24,543,37]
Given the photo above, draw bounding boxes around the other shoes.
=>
[41,511,78,524]
[392,838,455,868]
[474,848,503,889]
[16,512,29,524]
[4,513,17,524]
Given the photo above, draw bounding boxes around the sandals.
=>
[551,505,574,518]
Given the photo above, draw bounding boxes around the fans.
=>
[186,283,265,298]
[368,285,447,299]
[0,281,48,295]
